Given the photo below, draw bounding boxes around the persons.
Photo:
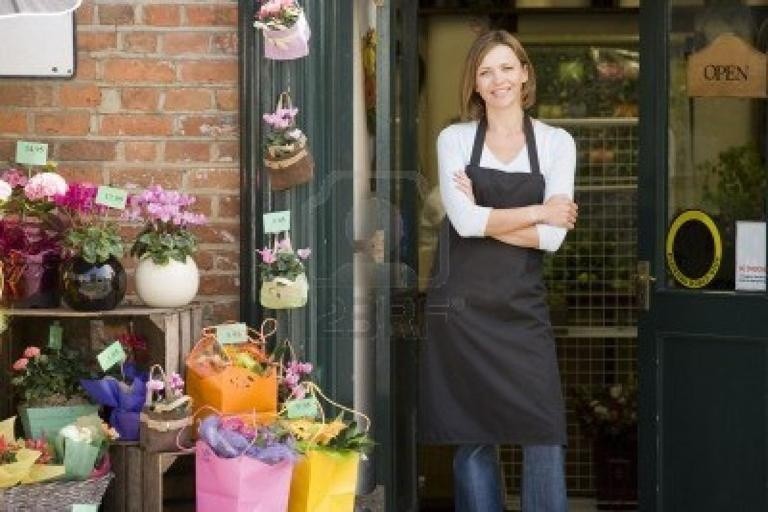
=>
[419,28,580,510]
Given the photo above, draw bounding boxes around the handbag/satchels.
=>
[279,379,371,512]
[175,404,292,512]
[186,317,279,415]
[139,363,195,454]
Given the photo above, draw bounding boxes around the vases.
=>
[0,250,201,308]
[592,433,640,502]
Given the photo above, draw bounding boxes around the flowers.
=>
[253,0,303,31]
[0,158,209,268]
[0,321,379,485]
[260,106,308,162]
[572,382,639,445]
[253,239,313,282]
